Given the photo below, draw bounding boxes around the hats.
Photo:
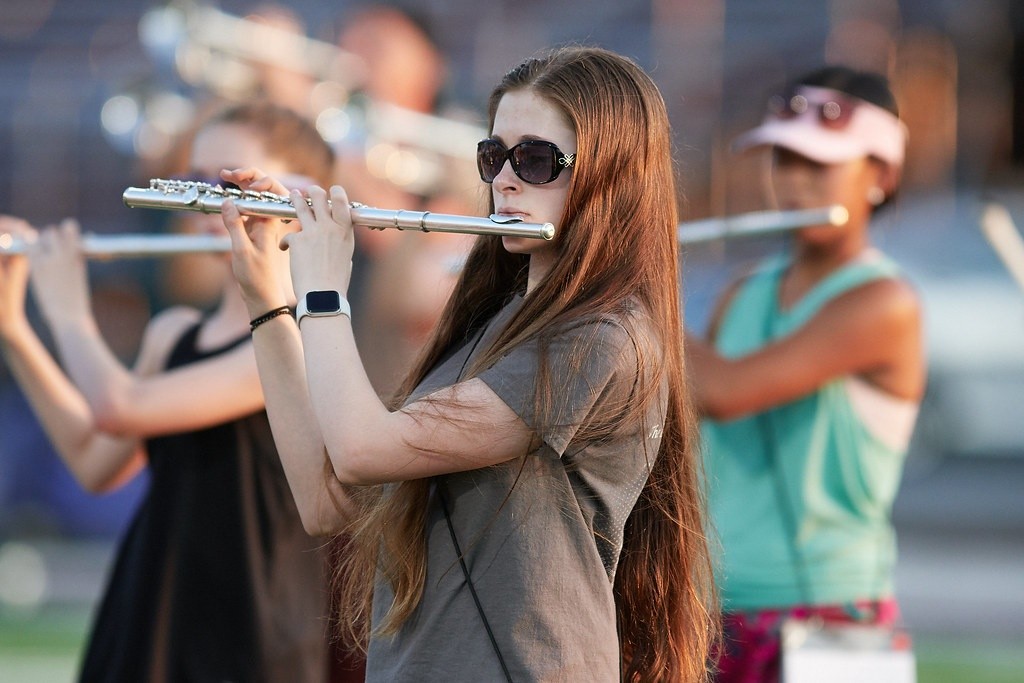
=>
[733,79,908,167]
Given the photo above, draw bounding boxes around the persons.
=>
[0,0,492,683]
[219,46,719,683]
[678,62,929,683]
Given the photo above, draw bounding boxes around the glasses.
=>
[764,88,861,131]
[476,138,576,185]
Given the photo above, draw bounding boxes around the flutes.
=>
[674,206,852,244]
[0,231,233,255]
[116,175,557,244]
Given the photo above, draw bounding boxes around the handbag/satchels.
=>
[780,617,918,683]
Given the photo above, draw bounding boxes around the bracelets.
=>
[250,305,294,325]
[249,311,296,331]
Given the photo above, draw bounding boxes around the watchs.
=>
[295,287,352,331]
